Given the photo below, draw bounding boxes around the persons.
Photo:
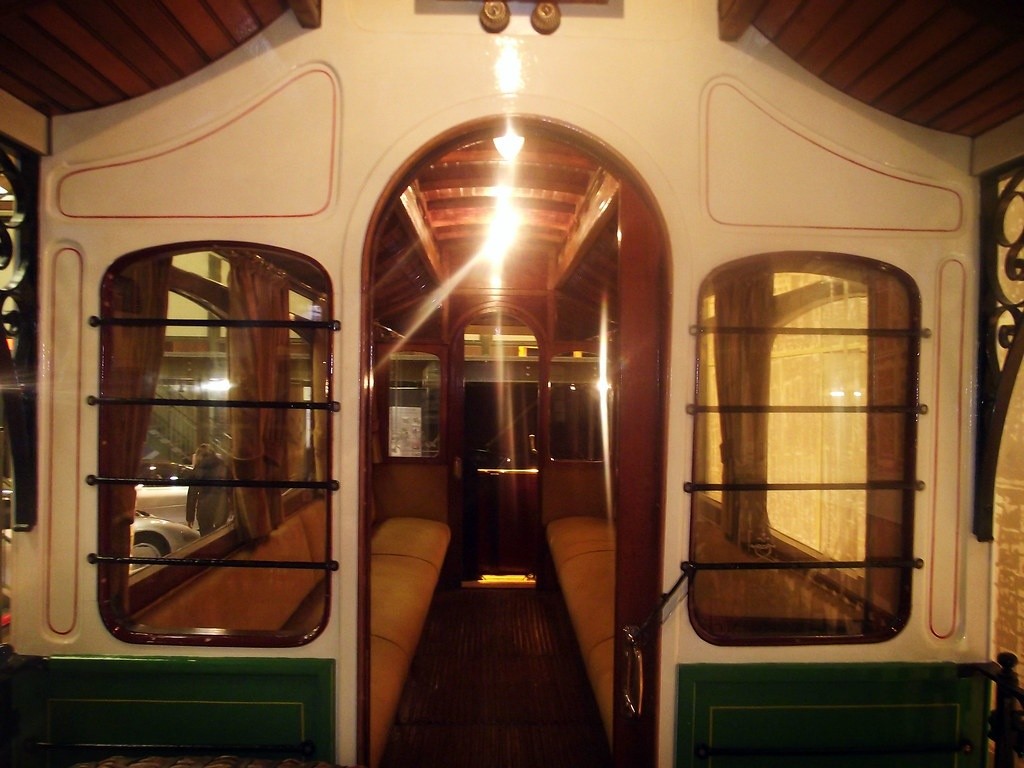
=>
[185,443,234,538]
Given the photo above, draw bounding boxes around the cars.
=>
[130,460,235,571]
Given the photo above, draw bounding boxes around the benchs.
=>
[547,481,886,768]
[80,468,452,768]
